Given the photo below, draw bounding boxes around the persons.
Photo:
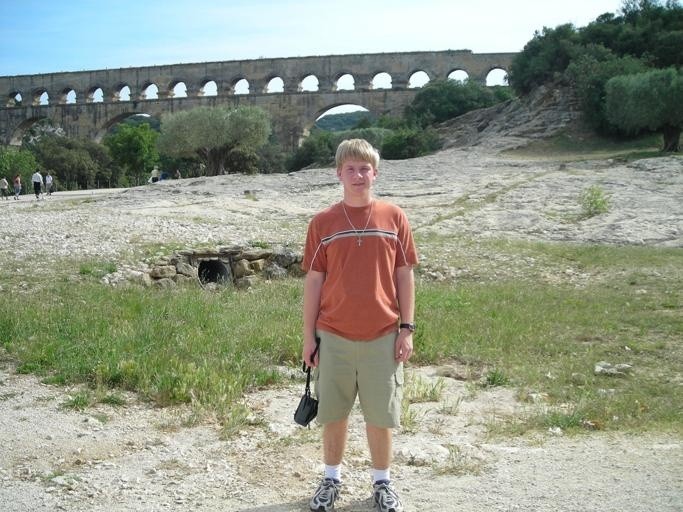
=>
[0,168,53,200]
[301,137,420,512]
[148,165,182,183]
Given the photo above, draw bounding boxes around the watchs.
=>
[400,323,415,332]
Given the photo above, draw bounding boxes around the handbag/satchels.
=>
[293,394,319,427]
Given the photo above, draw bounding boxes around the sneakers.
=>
[372,479,404,512]
[309,475,342,512]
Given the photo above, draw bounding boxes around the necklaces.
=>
[341,199,373,247]
